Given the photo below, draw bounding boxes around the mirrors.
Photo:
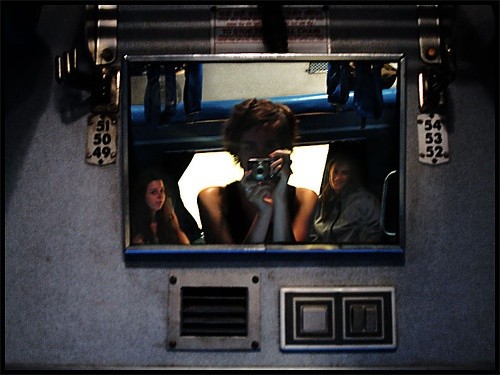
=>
[120,51,407,254]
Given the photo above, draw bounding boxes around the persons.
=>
[313,157,382,246]
[130,172,192,245]
[197,97,318,246]
